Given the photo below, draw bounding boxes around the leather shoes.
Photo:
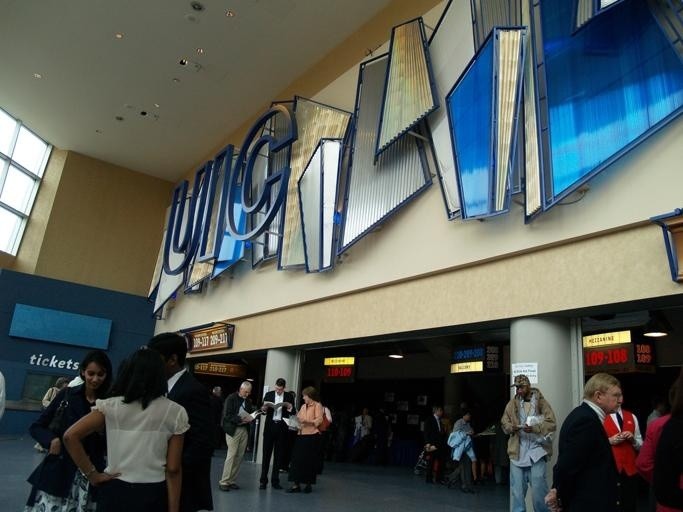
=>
[259,482,265,489]
[230,483,239,489]
[272,483,282,489]
[286,486,300,492]
[218,485,229,492]
[303,485,312,493]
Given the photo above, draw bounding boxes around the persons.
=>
[274,390,387,475]
[422,404,493,494]
[500,374,557,511]
[206,386,261,456]
[217,380,260,493]
[646,400,668,425]
[284,384,323,494]
[34,376,69,452]
[61,349,190,511]
[258,378,297,491]
[145,332,219,510]
[635,373,682,512]
[543,372,623,511]
[23,350,112,511]
[601,386,644,511]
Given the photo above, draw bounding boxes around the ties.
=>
[616,411,622,429]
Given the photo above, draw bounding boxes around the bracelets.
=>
[80,465,96,480]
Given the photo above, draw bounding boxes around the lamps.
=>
[643,313,670,340]
[386,340,406,361]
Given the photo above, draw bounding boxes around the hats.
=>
[509,374,529,387]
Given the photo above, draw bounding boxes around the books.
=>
[237,404,263,423]
[263,399,288,410]
[281,414,303,431]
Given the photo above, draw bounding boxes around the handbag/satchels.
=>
[48,387,70,432]
[313,403,330,432]
